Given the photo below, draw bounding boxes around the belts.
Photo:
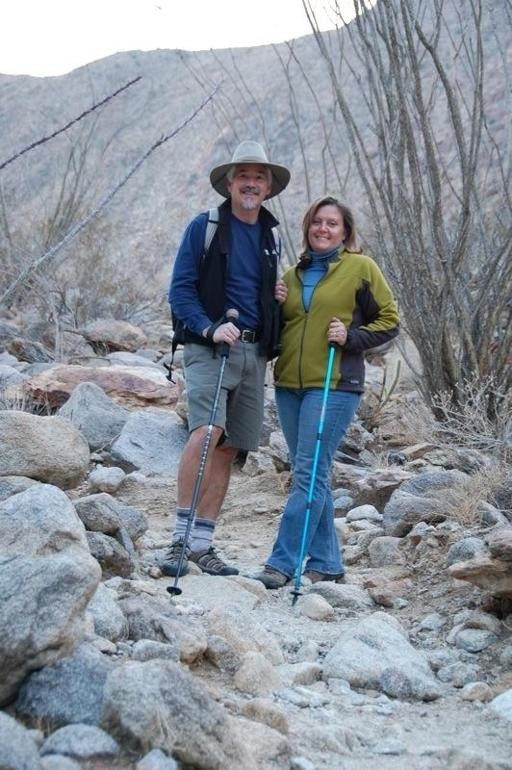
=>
[235,329,260,344]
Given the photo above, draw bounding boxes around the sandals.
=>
[187,545,239,576]
[161,537,191,577]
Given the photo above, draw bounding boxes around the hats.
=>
[209,140,291,200]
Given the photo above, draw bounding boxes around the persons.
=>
[151,141,290,583]
[254,192,397,590]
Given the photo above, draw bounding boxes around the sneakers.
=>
[255,566,288,587]
[298,566,344,587]
